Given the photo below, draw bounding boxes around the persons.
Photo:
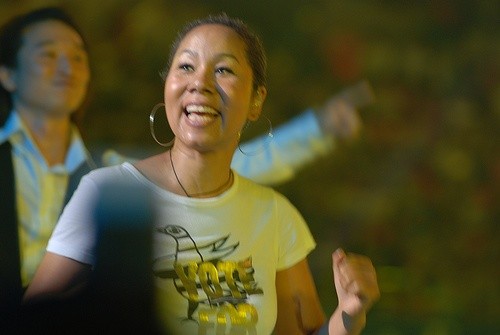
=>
[21,14,382,335]
[0,6,102,321]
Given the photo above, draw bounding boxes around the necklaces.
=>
[169,142,232,199]
